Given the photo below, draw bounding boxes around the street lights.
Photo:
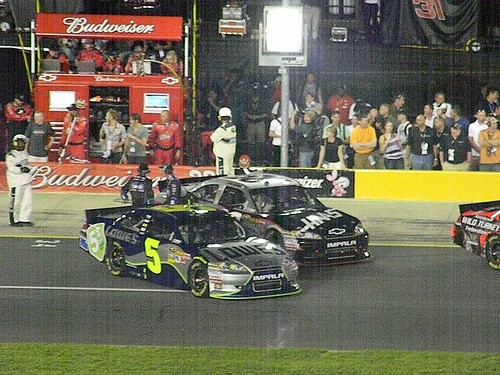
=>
[258,0,309,167]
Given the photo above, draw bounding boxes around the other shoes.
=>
[22,222,34,226]
[9,221,22,227]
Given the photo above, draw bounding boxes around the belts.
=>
[156,144,175,151]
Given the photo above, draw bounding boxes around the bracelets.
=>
[361,143,363,147]
[434,157,438,161]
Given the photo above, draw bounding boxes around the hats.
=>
[450,122,462,129]
[137,161,150,173]
[157,163,173,175]
[395,107,408,115]
[14,92,25,101]
[484,116,499,127]
[67,103,78,110]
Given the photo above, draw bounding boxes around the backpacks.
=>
[303,122,323,150]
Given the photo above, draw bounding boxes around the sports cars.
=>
[450,200,500,272]
[157,171,371,268]
[79,198,303,301]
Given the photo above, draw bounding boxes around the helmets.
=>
[218,107,233,121]
[12,134,29,150]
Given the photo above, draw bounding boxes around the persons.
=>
[41,35,184,75]
[159,164,181,205]
[5,134,38,226]
[193,224,212,243]
[210,107,237,177]
[0,66,500,173]
[121,163,155,206]
[276,190,302,211]
